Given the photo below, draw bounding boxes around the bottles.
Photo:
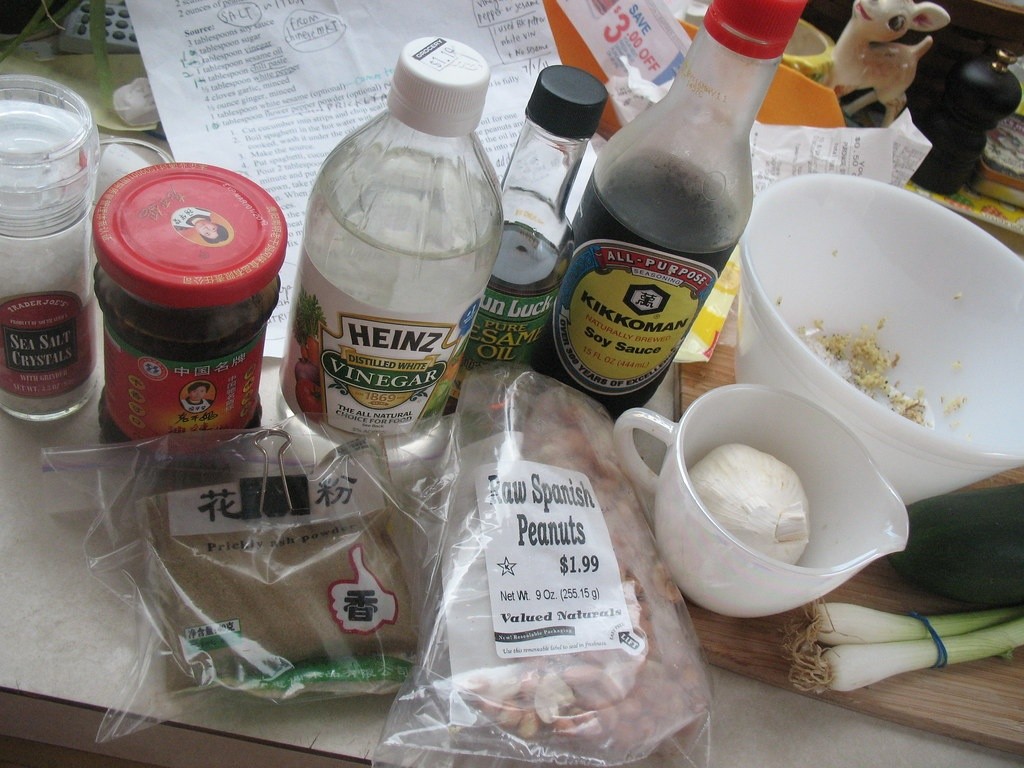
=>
[280,32,511,461]
[428,64,610,425]
[93,155,289,476]
[535,0,817,418]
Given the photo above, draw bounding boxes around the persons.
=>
[181,381,215,414]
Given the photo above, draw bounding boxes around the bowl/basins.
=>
[734,174,1023,514]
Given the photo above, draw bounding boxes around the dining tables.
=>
[3,4,1024,768]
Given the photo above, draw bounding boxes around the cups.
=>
[1,70,102,422]
[614,379,911,618]
